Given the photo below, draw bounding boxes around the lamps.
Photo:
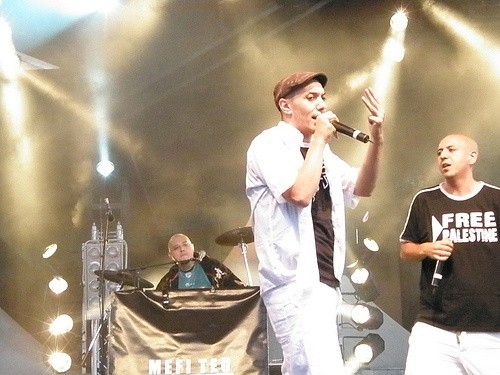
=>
[344,206,385,367]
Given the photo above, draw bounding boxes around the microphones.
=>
[329,119,374,143]
[198,250,206,262]
[104,198,114,221]
[430,260,444,296]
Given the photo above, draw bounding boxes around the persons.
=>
[245,70,388,375]
[398,134,500,375]
[154,232,245,299]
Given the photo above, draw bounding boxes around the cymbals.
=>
[214,226,255,246]
[92,270,154,288]
